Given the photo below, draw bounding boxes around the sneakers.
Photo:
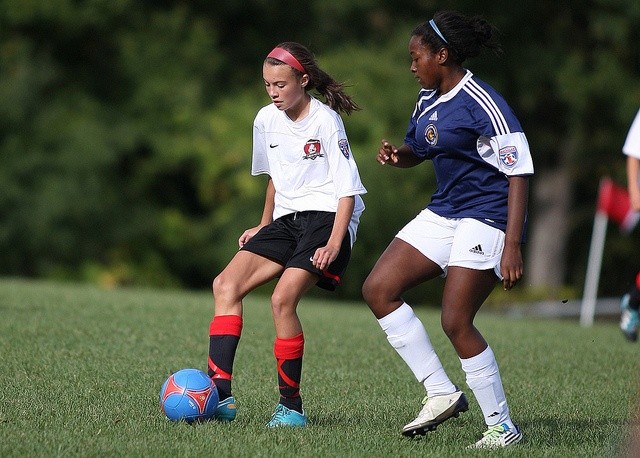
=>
[265,404,307,429]
[464,424,526,452]
[216,396,238,420]
[621,292,639,339]
[400,387,471,439]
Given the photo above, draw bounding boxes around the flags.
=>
[597,176,639,235]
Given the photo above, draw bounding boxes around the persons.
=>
[361,8,533,452]
[206,41,367,428]
[621,111,639,338]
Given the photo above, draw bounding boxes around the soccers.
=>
[160,368,219,424]
